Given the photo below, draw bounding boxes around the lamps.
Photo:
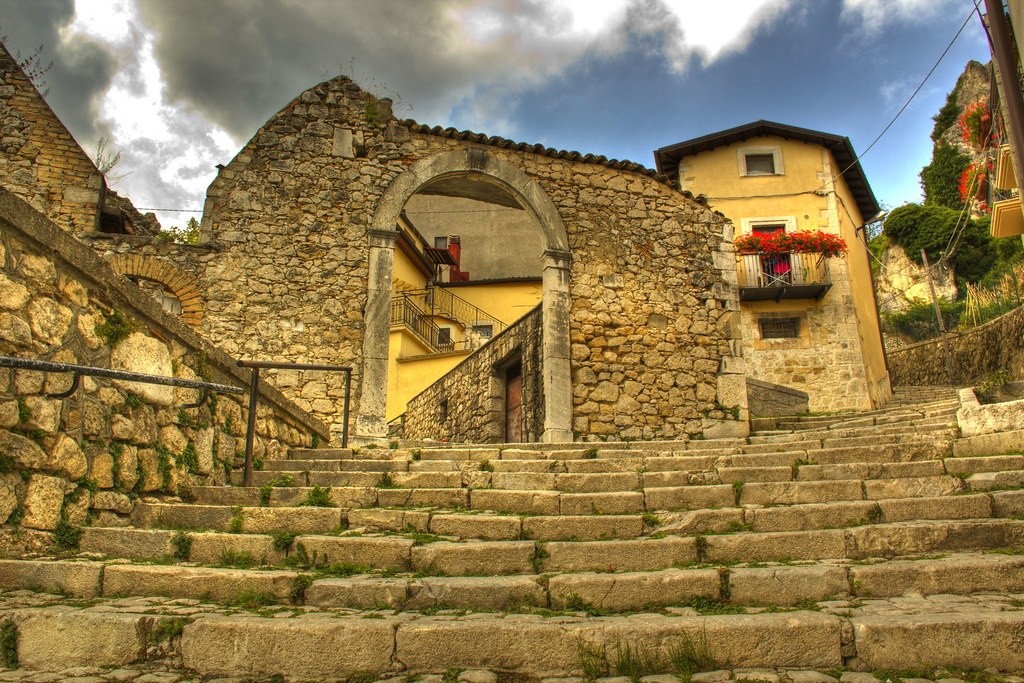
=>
[854,209,888,237]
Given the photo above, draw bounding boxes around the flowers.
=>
[955,101,992,151]
[733,228,849,258]
[955,162,982,201]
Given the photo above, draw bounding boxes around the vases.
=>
[977,174,986,202]
[977,112,991,148]
